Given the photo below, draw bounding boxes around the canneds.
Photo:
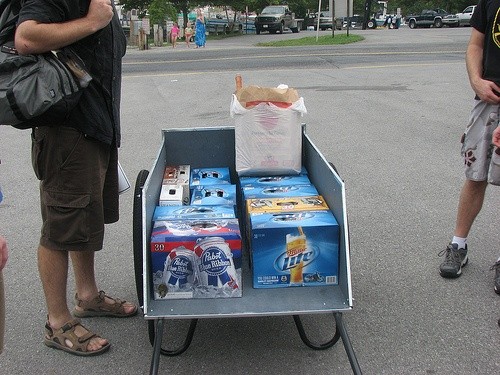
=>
[163,247,200,292]
[193,236,240,293]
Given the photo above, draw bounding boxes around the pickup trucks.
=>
[406,4,478,28]
[254,5,304,34]
[305,10,388,30]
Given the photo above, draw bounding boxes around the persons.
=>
[13,1,137,356]
[437,1,500,294]
[192,8,206,49]
[185,23,193,48]
[170,22,180,49]
[384,15,401,29]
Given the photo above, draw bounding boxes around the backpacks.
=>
[0,0,92,129]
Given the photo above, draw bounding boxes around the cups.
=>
[286,234,306,284]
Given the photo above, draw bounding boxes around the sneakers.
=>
[437,240,469,278]
[489,257,500,294]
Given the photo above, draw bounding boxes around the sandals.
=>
[74,290,138,318]
[44,314,111,357]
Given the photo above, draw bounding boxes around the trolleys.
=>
[134,124,363,375]
